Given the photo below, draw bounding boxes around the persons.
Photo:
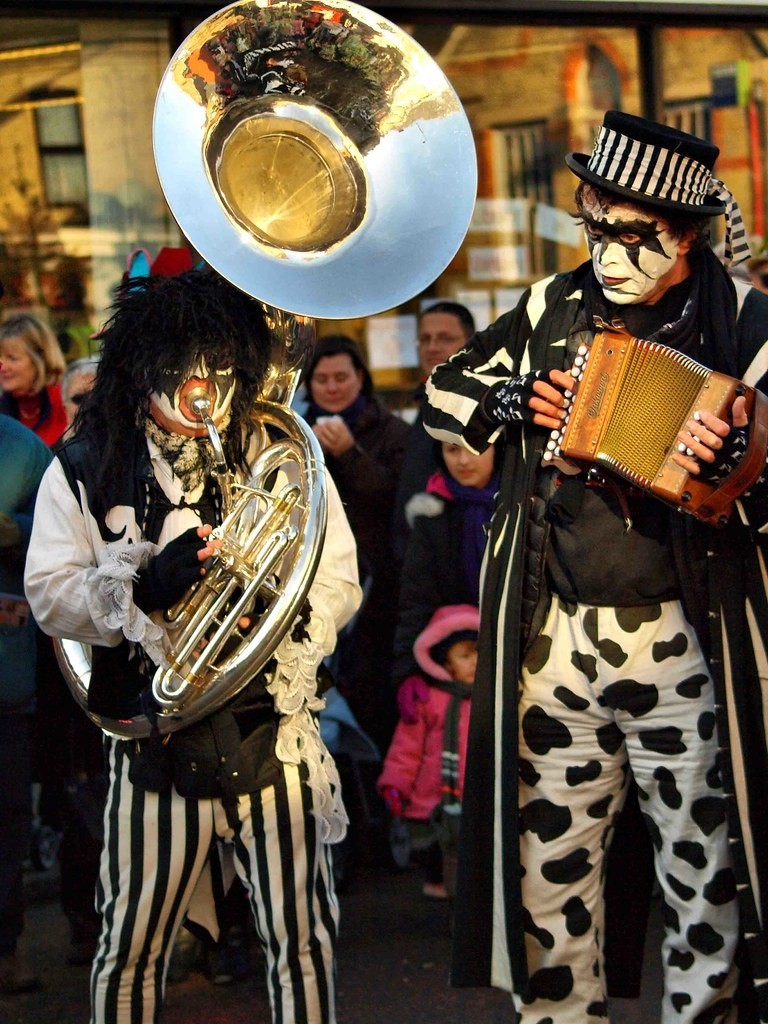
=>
[23,268,369,1024]
[417,104,768,1023]
[0,299,520,997]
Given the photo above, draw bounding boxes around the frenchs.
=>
[44,0,479,742]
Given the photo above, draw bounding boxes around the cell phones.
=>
[316,416,344,426]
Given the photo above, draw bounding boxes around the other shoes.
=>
[56,926,96,969]
[0,952,39,992]
[423,880,448,901]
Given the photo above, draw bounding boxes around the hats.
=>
[564,109,752,271]
